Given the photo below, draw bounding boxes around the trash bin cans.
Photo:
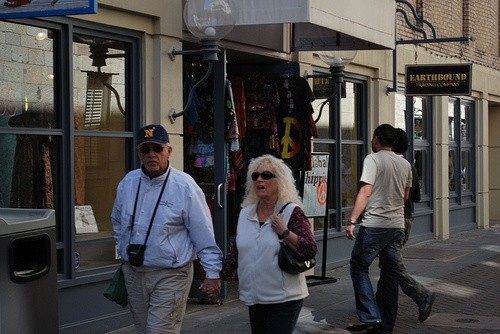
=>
[0,208,58,334]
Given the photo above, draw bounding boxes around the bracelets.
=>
[281,229,289,238]
[348,221,356,226]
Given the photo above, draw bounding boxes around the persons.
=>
[199,153,317,334]
[345,124,437,334]
[111,124,223,334]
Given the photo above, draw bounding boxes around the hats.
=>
[136,124,169,149]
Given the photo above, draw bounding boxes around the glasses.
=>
[251,171,276,181]
[138,145,168,153]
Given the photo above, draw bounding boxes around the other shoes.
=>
[418,290,435,322]
[351,320,382,333]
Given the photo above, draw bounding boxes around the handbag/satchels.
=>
[103,261,130,307]
[276,201,317,273]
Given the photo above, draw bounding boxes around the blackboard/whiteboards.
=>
[300,153,330,218]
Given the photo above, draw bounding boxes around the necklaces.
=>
[262,214,265,220]
